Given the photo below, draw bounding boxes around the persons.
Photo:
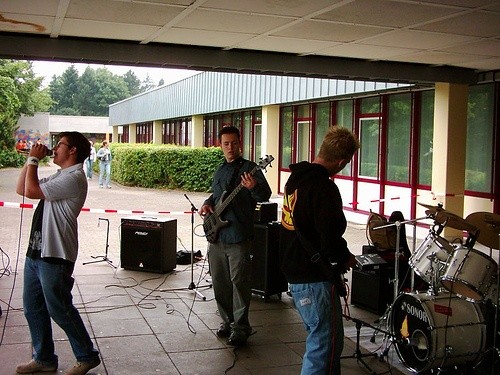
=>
[16,130,103,375]
[279,125,359,375]
[96,141,112,189]
[198,125,272,347]
[85,140,97,179]
[16,137,41,151]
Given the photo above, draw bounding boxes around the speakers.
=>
[249,221,288,301]
[120,215,178,274]
[351,244,405,315]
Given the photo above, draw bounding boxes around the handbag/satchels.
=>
[366,211,407,250]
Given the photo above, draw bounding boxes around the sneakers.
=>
[65,360,101,375]
[16,359,57,373]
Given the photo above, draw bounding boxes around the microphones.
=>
[18,148,53,156]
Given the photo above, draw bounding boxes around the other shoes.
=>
[107,185,112,189]
[100,185,103,188]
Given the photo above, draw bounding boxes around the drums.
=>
[390,289,487,374]
[408,231,456,289]
[439,245,498,303]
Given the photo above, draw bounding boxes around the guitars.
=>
[202,154,275,244]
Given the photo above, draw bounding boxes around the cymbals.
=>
[417,201,478,232]
[465,211,500,250]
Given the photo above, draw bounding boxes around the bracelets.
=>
[27,156,39,168]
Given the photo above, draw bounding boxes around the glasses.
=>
[56,141,72,148]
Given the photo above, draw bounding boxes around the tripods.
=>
[342,314,406,375]
[159,194,212,300]
[81,217,117,269]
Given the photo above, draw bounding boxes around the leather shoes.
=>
[226,329,251,345]
[216,324,231,336]
